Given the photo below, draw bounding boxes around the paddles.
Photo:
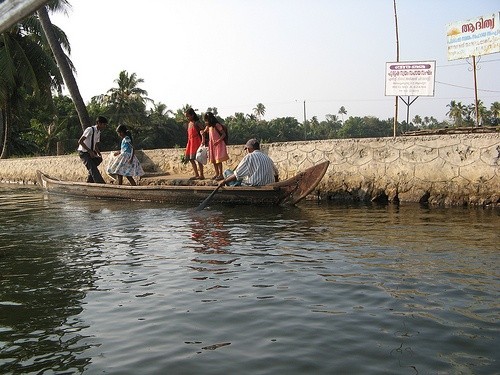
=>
[196,185,220,211]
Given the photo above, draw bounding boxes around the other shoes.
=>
[216,175,224,180]
[197,175,205,179]
[195,174,200,179]
[212,176,217,179]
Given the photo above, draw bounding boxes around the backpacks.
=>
[214,121,228,145]
[195,121,209,144]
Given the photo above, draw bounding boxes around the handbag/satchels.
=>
[196,144,207,165]
[92,149,102,166]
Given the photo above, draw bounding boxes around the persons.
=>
[223,138,279,186]
[78,116,107,183]
[200,112,226,180]
[184,107,206,179]
[107,124,146,186]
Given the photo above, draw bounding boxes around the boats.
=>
[35,159,335,207]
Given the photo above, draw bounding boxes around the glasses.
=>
[244,147,247,150]
